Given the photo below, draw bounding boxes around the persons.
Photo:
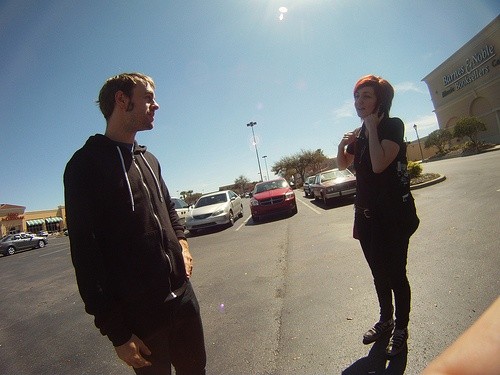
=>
[63,73,206,375]
[338,75,420,355]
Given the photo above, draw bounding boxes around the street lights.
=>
[246,121,264,182]
[262,156,269,180]
[413,125,425,163]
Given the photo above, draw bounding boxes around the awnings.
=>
[45,217,63,223]
[26,219,45,226]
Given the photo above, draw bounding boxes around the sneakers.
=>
[363,319,394,340]
[385,324,408,355]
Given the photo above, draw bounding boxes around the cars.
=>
[185,190,243,234]
[171,197,193,227]
[249,178,297,222]
[302,167,358,206]
[0,233,48,255]
[14,230,48,239]
